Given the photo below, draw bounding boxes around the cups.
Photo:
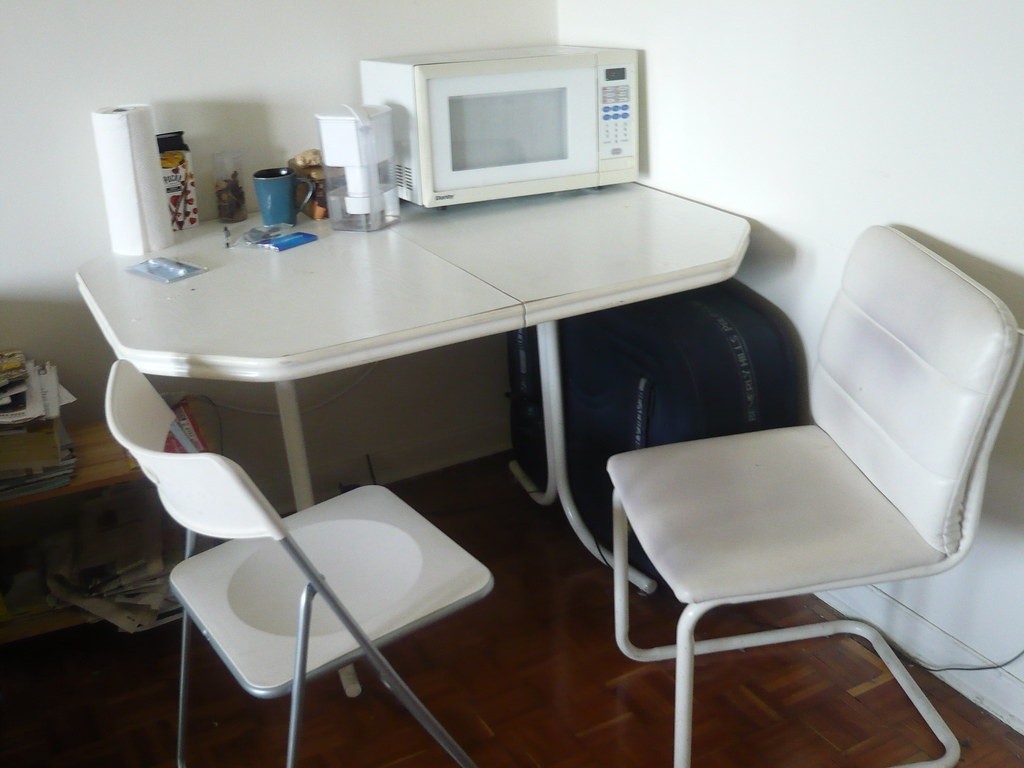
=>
[252,166,315,226]
[214,150,248,223]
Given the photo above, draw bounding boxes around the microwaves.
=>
[359,45,639,210]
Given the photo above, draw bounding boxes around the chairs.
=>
[612,226,1024,768]
[103,358,496,768]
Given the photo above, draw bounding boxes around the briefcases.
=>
[510,278,800,577]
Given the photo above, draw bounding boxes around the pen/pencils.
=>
[223,226,230,248]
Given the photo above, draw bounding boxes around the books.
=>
[41,488,185,635]
[1,348,80,502]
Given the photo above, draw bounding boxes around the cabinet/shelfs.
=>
[0,419,224,641]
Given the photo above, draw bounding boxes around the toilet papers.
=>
[86,103,175,258]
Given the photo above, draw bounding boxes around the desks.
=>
[72,183,751,594]
